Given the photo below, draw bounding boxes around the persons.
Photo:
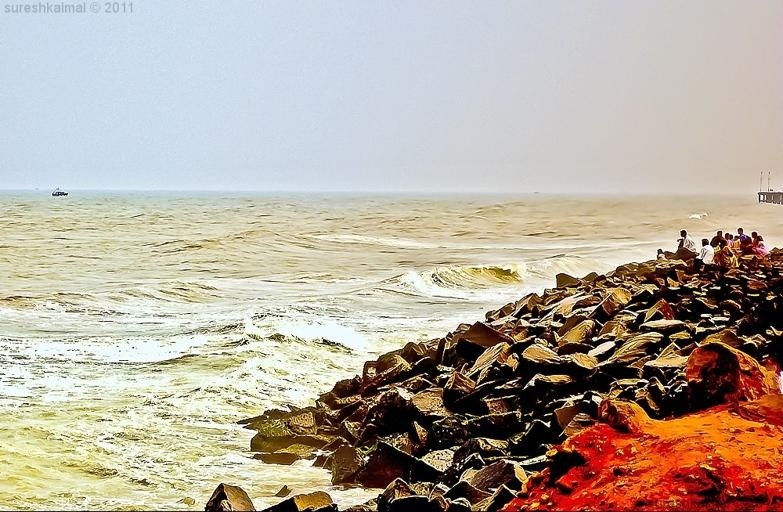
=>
[709,228,768,269]
[693,239,714,273]
[676,229,696,253]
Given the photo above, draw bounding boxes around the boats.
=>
[52,191,68,196]
